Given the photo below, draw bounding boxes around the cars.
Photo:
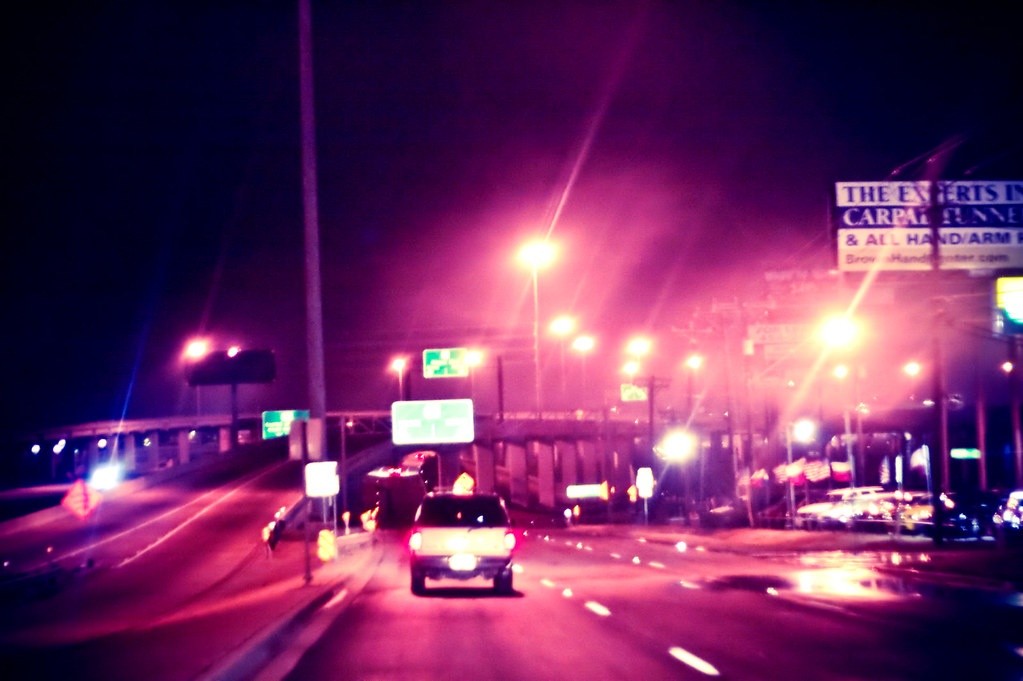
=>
[831,492,925,535]
[993,491,1023,538]
[916,491,998,540]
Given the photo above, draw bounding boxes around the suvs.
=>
[406,491,516,594]
[794,487,883,529]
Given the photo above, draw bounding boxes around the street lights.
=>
[630,330,656,480]
[513,229,559,419]
